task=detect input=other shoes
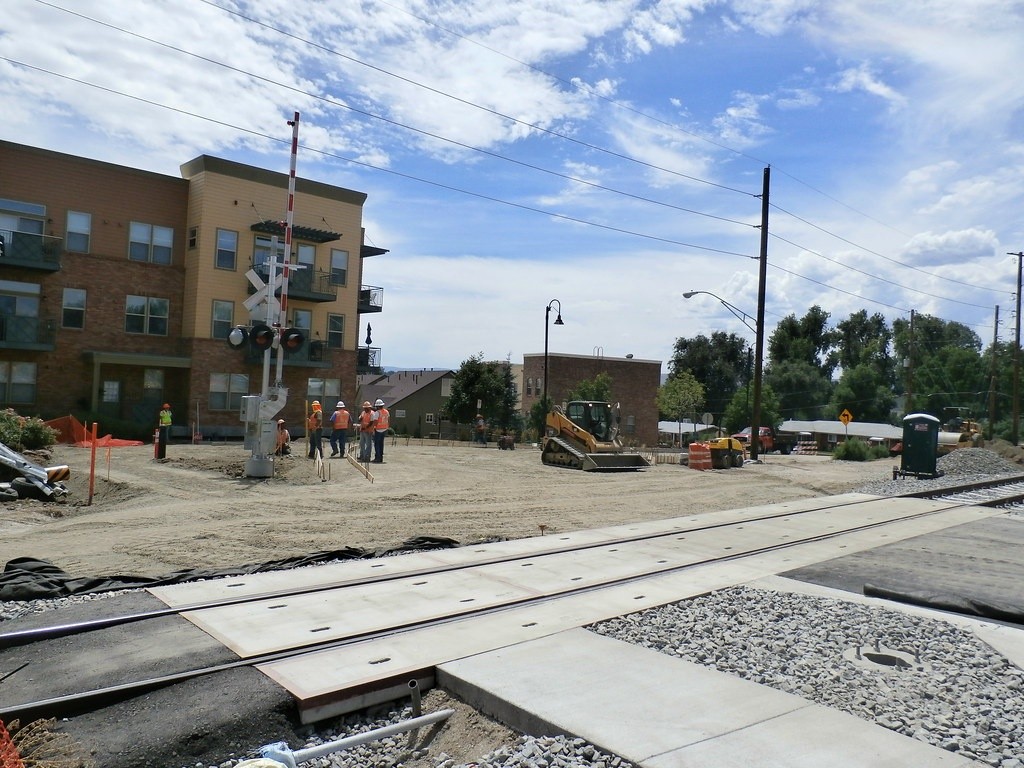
[331,448,338,456]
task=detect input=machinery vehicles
[936,417,985,457]
[540,399,653,472]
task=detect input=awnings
[251,220,342,243]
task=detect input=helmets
[163,403,170,408]
[278,419,285,424]
[335,401,345,407]
[362,401,372,408]
[312,400,321,406]
[374,399,385,406]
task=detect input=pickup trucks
[729,426,797,455]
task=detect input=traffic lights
[250,324,274,351]
[281,327,306,354]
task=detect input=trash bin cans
[892,414,940,480]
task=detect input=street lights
[682,290,765,461]
[541,298,564,437]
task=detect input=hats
[475,414,483,418]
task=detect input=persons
[972,430,981,447]
[474,414,487,444]
[276,419,291,455]
[330,401,353,458]
[308,400,324,459]
[159,404,172,444]
[358,398,391,463]
